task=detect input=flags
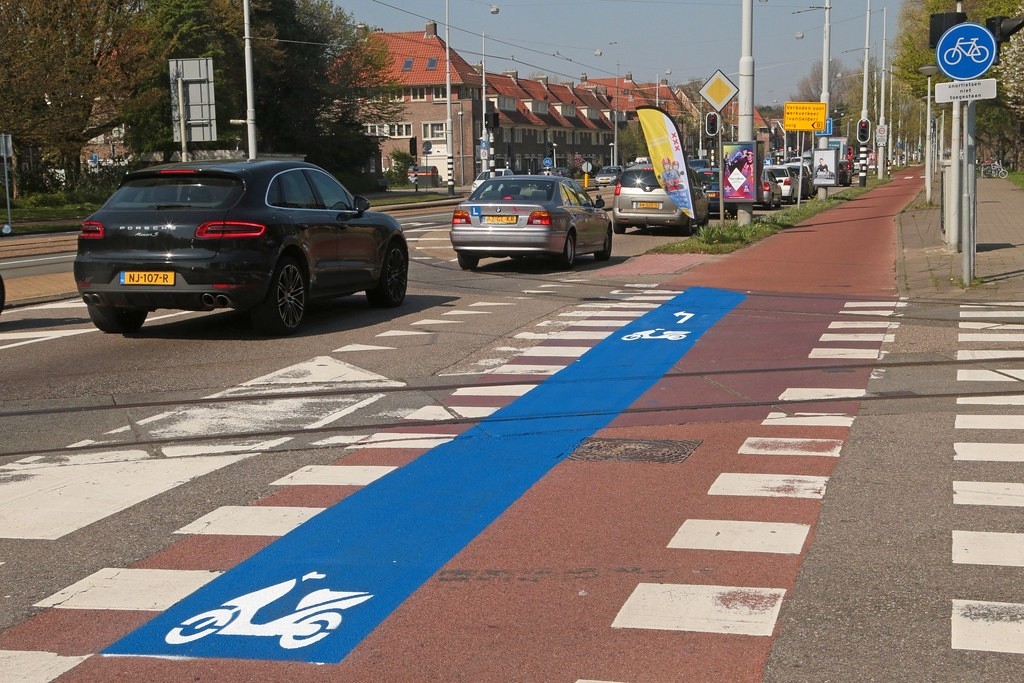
[636,105,695,220]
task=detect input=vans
[634,156,652,165]
[688,159,710,171]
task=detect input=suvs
[73,158,409,336]
[612,164,711,237]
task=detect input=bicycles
[975,156,1010,179]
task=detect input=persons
[817,158,828,172]
[662,157,684,190]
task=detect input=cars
[782,157,818,200]
[594,166,623,188]
[471,168,513,199]
[537,167,575,179]
[839,161,852,187]
[855,157,860,169]
[696,168,737,217]
[763,164,799,205]
[759,168,783,210]
[450,174,613,271]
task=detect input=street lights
[931,102,950,174]
[609,142,614,166]
[918,65,940,205]
[553,143,558,169]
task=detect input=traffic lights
[986,16,1024,42]
[857,118,871,144]
[706,112,719,137]
[847,147,853,158]
